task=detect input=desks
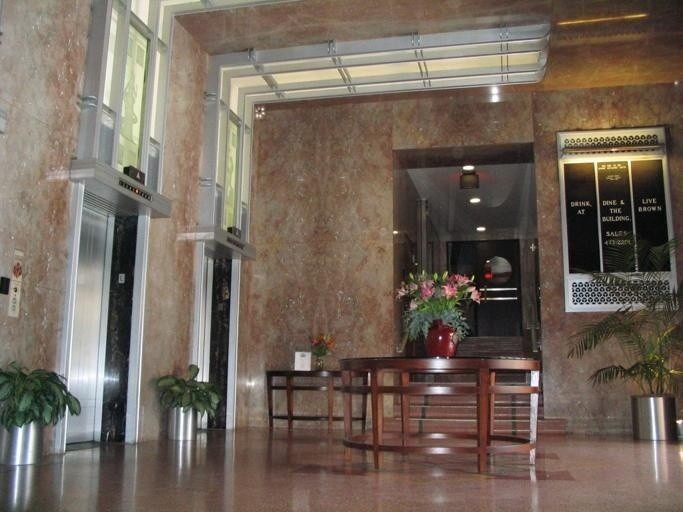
[337,354,547,476]
[261,363,377,435]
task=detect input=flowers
[308,333,335,358]
[394,269,488,345]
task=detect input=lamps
[457,164,482,192]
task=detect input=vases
[313,352,326,372]
[422,317,464,362]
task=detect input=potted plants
[0,360,83,470]
[151,362,222,443]
[560,231,683,444]
